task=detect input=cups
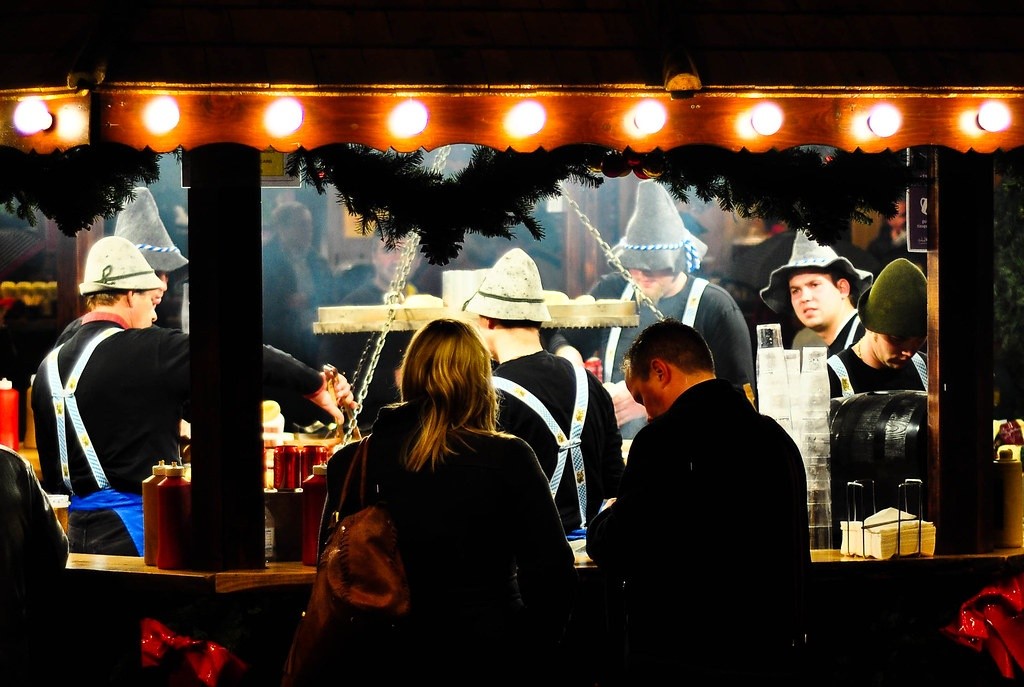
[755,322,832,559]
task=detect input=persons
[585,317,824,687]
[462,247,624,536]
[826,258,927,400]
[0,447,69,687]
[317,320,581,687]
[760,229,873,374]
[548,179,756,439]
[324,230,424,436]
[30,188,359,556]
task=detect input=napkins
[839,506,936,560]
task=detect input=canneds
[273,445,329,493]
[584,357,602,383]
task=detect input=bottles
[142,460,191,570]
[24,374,38,449]
[0,378,19,452]
[993,449,1023,549]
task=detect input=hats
[79,237,168,294]
[857,256,930,334]
[114,187,190,272]
[760,229,873,313]
[462,247,552,324]
[610,179,709,270]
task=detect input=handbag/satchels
[282,505,411,686]
